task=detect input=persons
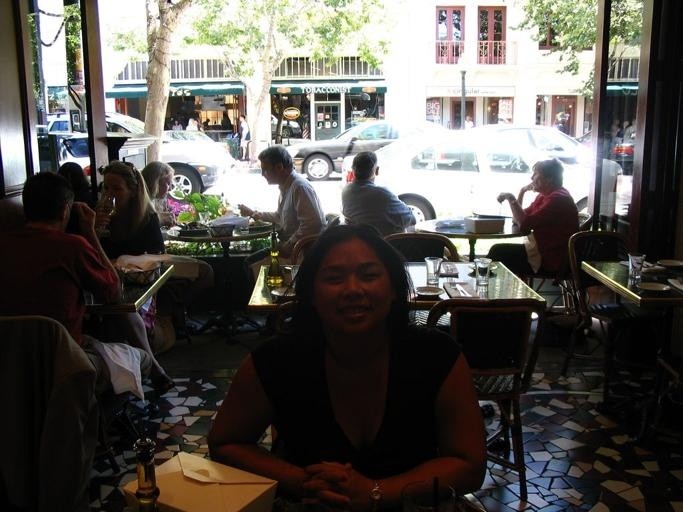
[207,222,488,512]
[341,151,416,239]
[172,120,183,130]
[464,115,474,128]
[237,147,327,314]
[610,118,635,146]
[238,115,250,161]
[0,158,178,428]
[556,119,568,134]
[483,158,579,280]
[185,118,198,131]
[203,119,210,127]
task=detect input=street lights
[458,53,469,129]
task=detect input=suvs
[270,113,302,140]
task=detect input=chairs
[1,211,648,345]
[557,230,668,419]
[427,297,550,502]
[1,314,142,476]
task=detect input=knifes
[455,283,472,297]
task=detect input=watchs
[368,480,383,512]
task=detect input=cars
[47,113,229,200]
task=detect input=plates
[179,230,208,236]
[635,260,683,293]
[468,264,498,271]
[271,288,295,297]
[414,287,444,297]
[249,224,272,231]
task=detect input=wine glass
[95,193,115,239]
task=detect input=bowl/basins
[211,224,235,238]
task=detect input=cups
[424,256,443,284]
[627,253,647,278]
[627,278,642,294]
[198,212,210,226]
[474,258,492,286]
[240,219,249,235]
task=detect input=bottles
[133,438,161,511]
[265,227,284,288]
